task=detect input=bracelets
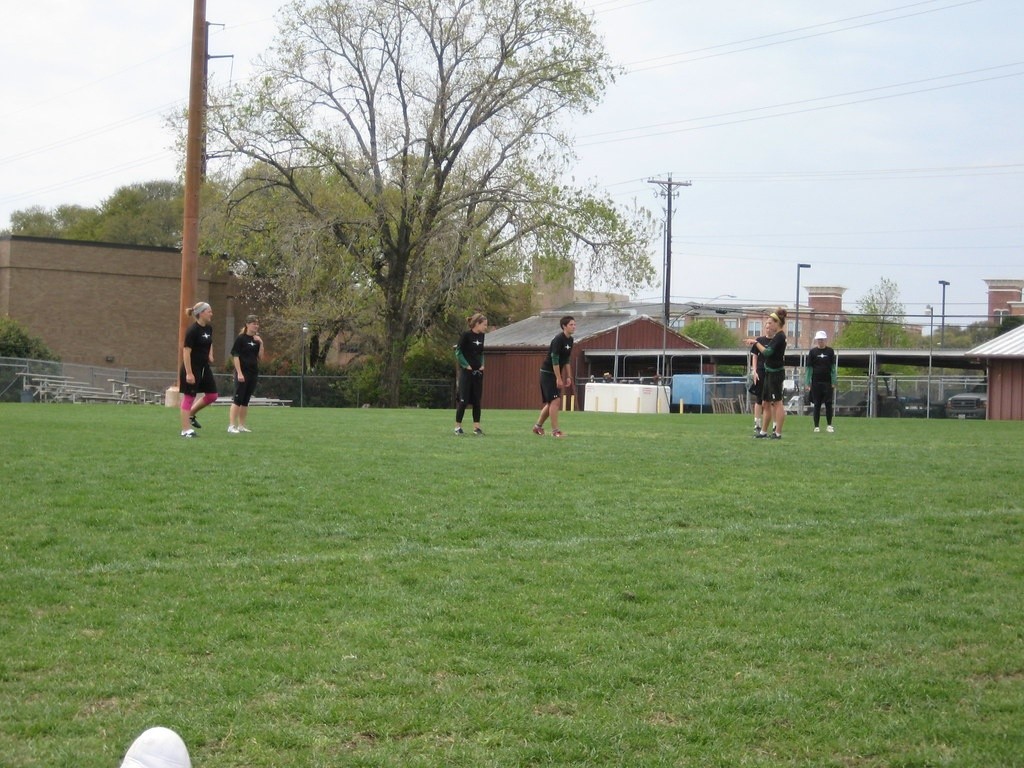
[754,341,758,345]
[567,376,573,378]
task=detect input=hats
[246,315,258,323]
[815,331,827,339]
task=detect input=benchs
[209,399,294,408]
[15,372,165,406]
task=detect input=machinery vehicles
[854,371,933,419]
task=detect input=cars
[944,382,986,420]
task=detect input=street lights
[795,263,812,396]
[938,281,950,400]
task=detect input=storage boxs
[583,383,670,414]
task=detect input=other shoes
[827,426,834,432]
[533,426,544,435]
[455,428,464,435]
[228,425,239,432]
[762,433,781,440]
[814,428,820,432]
[550,431,561,437]
[189,415,201,428]
[753,433,767,439]
[182,429,196,435]
[238,425,251,432]
[473,428,485,435]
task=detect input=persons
[179,302,218,438]
[454,313,488,436]
[120,727,192,768]
[742,307,787,440]
[533,316,575,437]
[227,315,264,434]
[806,331,837,433]
